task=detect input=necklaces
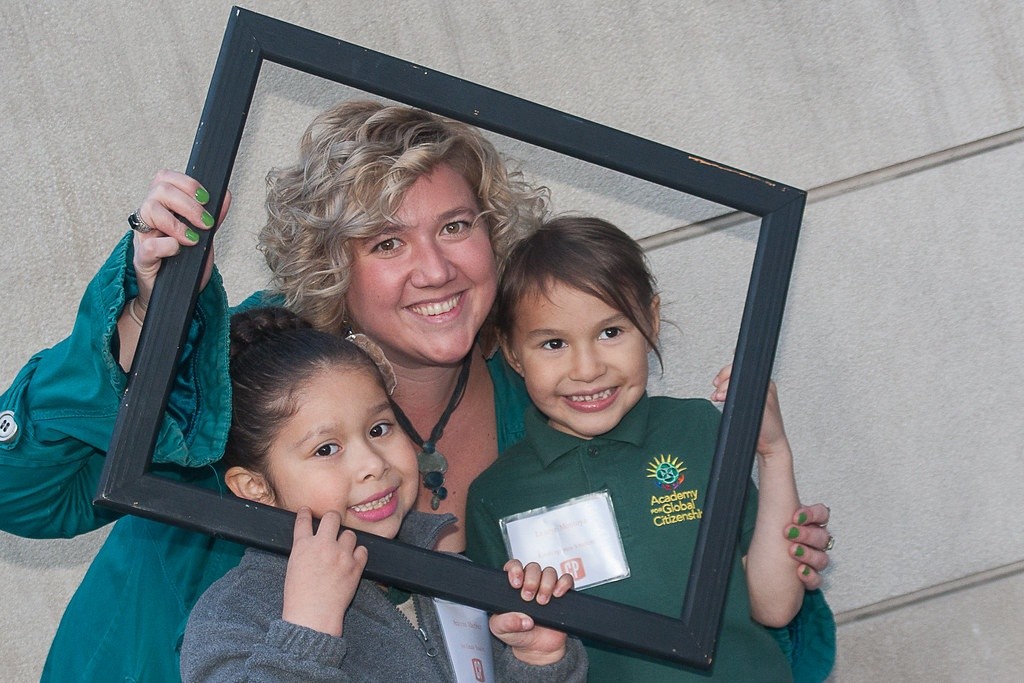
[385,350,472,513]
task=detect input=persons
[1,97,839,683]
[178,305,594,683]
[461,218,811,683]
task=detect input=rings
[821,536,834,552]
[815,506,830,527]
[128,208,156,234]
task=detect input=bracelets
[129,298,146,327]
